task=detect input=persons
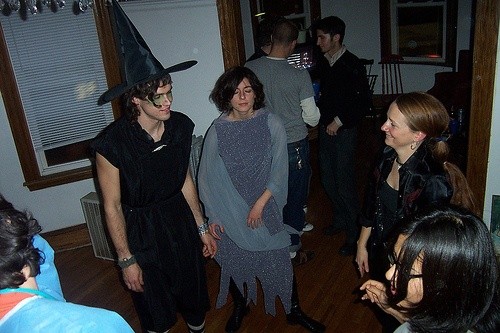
[360,211,500,333]
[199,66,326,333]
[0,193,135,333]
[315,15,373,256]
[92,0,217,333]
[354,92,480,304]
[246,20,321,267]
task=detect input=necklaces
[150,122,161,141]
[396,157,404,166]
[229,111,254,122]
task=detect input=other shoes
[292,250,313,267]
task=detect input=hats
[97,0,197,106]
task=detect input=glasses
[388,249,423,281]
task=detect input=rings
[252,220,254,223]
[358,266,363,270]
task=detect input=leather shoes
[324,223,344,233]
[337,234,359,255]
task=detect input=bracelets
[198,223,209,237]
[118,256,136,269]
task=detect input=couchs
[427,50,471,104]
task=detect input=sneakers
[302,221,313,231]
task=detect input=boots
[286,270,326,333]
[225,277,250,333]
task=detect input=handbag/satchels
[367,229,387,279]
